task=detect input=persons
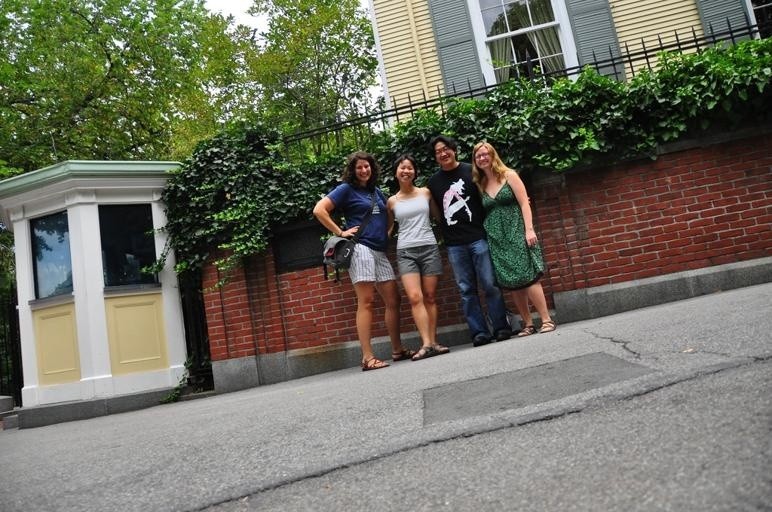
[383,155,450,361]
[424,136,531,346]
[312,151,417,370]
[469,141,556,336]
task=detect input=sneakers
[495,329,511,341]
[471,333,492,347]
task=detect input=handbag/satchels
[323,236,353,269]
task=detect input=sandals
[362,356,390,370]
[518,326,535,336]
[539,321,556,332]
[392,343,449,361]
